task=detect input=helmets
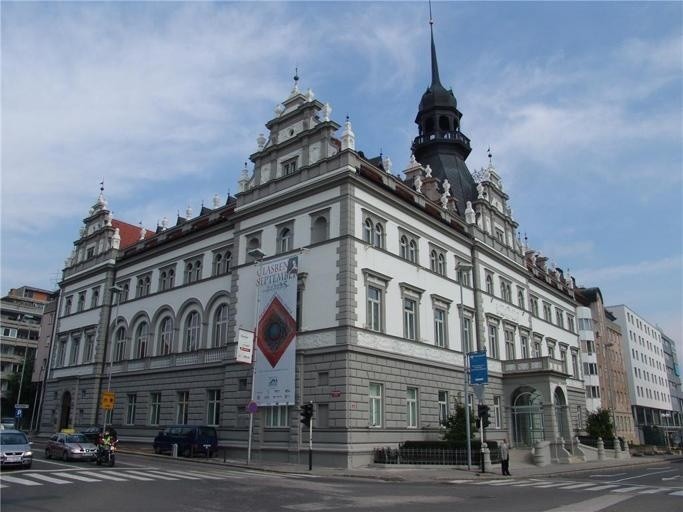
[104,431,109,436]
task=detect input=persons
[500,439,512,476]
[98,431,116,458]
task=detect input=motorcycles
[95,440,118,466]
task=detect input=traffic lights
[301,403,314,426]
[484,406,492,426]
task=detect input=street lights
[604,342,617,437]
[17,314,34,404]
[455,260,473,471]
[248,249,267,461]
[102,285,123,433]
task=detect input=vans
[153,424,218,459]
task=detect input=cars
[0,430,34,468]
[0,417,16,430]
[45,432,99,461]
[73,422,119,445]
